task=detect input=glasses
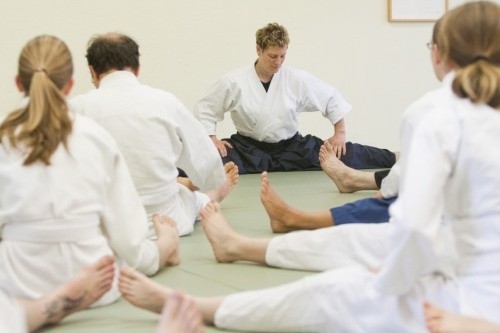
[426,40,433,49]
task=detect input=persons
[177,22,400,177]
[69,31,239,241]
[118,0,499,333]
[423,300,499,333]
[198,14,451,273]
[153,289,205,332]
[0,34,180,308]
[260,157,400,234]
[0,254,114,333]
[317,138,391,194]
